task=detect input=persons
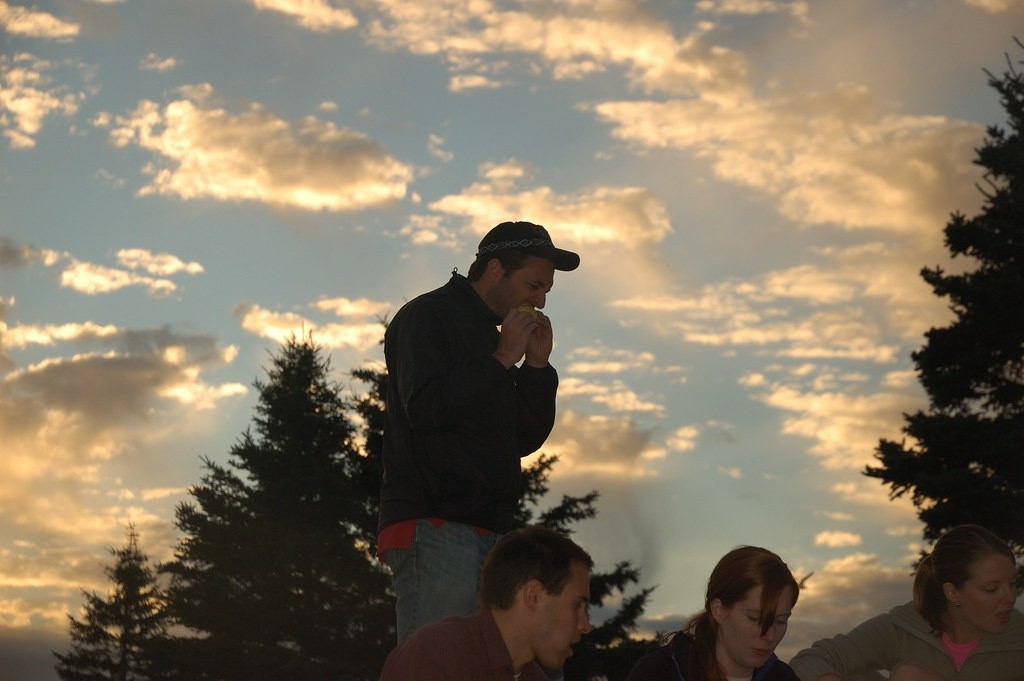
[791,523,1024,681]
[627,546,802,681]
[379,526,597,681]
[376,220,579,681]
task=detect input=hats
[477,221,580,271]
